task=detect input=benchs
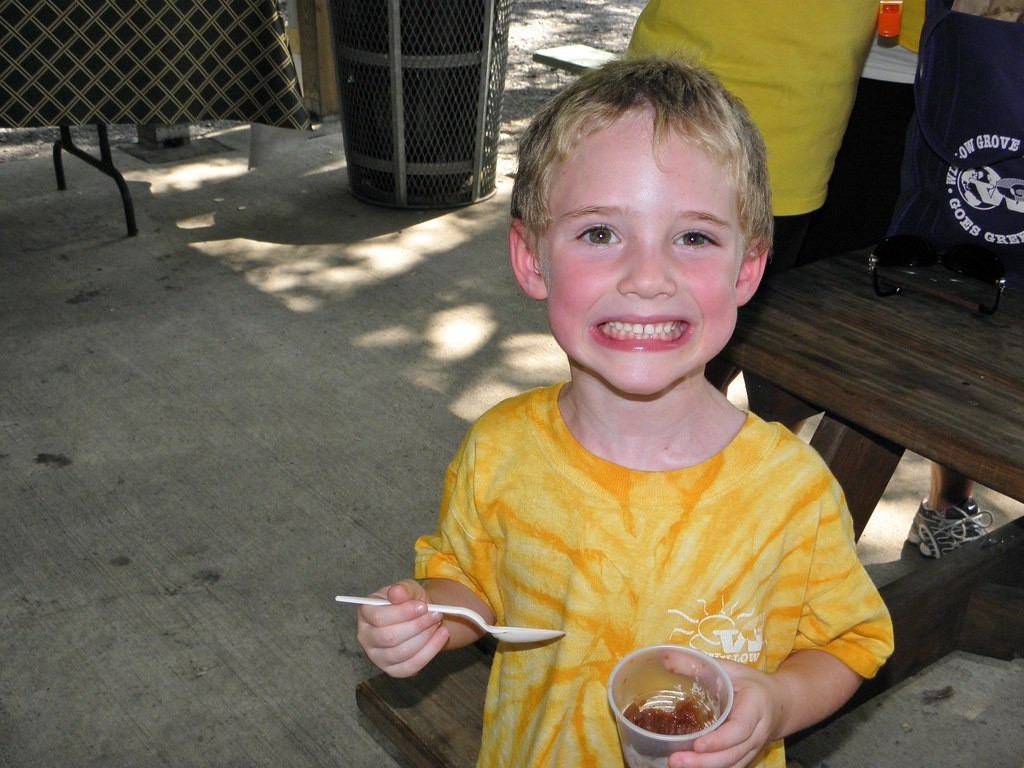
[531,44,619,73]
[356,632,500,768]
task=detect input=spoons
[335,595,566,643]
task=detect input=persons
[628,0,1024,558]
[356,58,894,768]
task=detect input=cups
[608,644,734,768]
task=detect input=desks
[0,0,314,238]
[704,241,1024,747]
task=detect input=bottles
[878,0,903,48]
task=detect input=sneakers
[907,496,993,561]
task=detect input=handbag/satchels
[887,11,1024,279]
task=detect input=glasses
[868,232,1007,316]
